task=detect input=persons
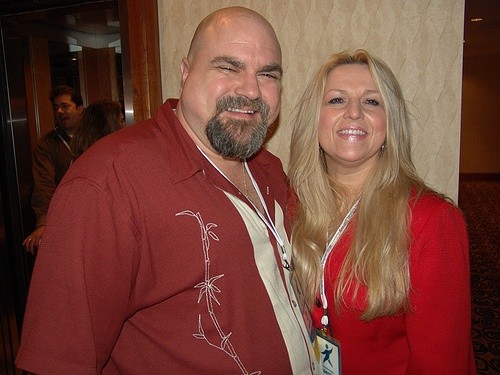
[22,83,127,257]
[14,6,320,375]
[287,49,476,375]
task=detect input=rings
[29,237,31,242]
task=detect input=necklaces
[241,159,250,199]
